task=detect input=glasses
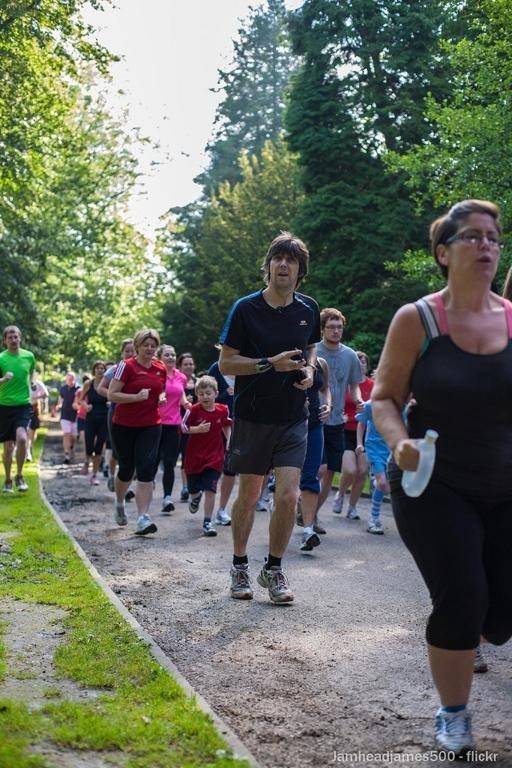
[321,324,346,332]
[439,232,506,252]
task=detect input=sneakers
[0,449,389,537]
[471,645,493,675]
[427,704,479,762]
[254,554,296,605]
[300,524,323,551]
[228,560,256,602]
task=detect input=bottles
[399,429,439,498]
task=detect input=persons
[370,199,512,758]
